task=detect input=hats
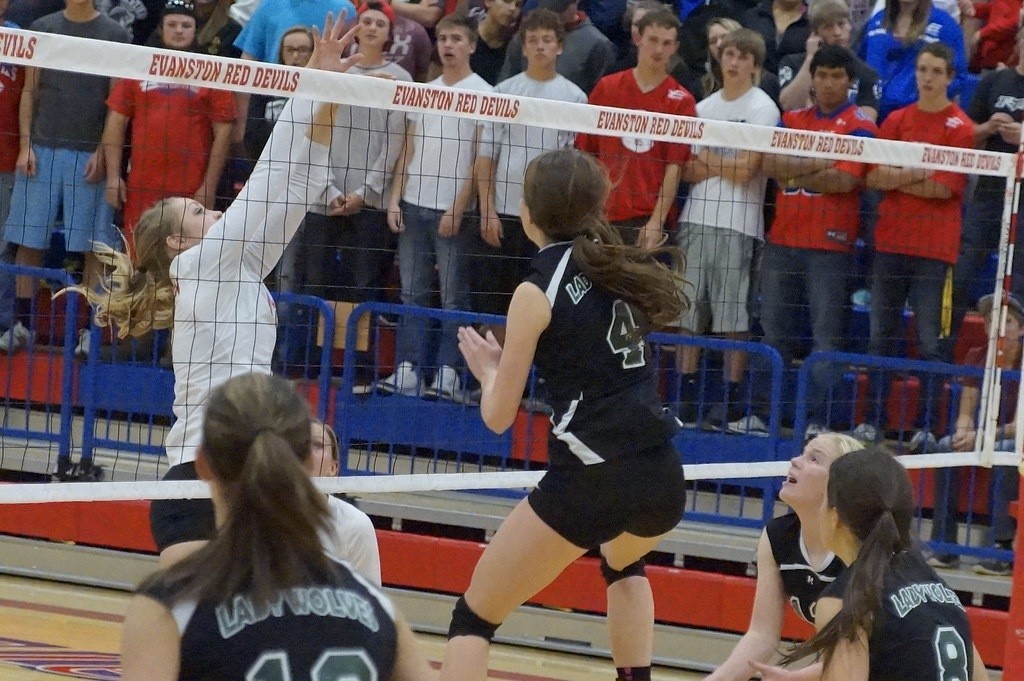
[161,0,199,20]
[979,290,1023,317]
[357,0,396,23]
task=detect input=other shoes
[351,372,375,393]
[727,414,771,438]
[101,334,153,361]
[425,365,459,401]
[804,424,829,441]
[160,344,173,367]
[377,360,425,398]
[665,402,700,429]
[909,429,937,453]
[702,403,729,432]
[852,422,886,447]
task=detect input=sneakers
[0,320,37,355]
[75,329,91,357]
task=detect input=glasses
[283,45,311,57]
[528,35,555,45]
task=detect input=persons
[305,417,381,592]
[52,7,366,568]
[119,371,435,681]
[923,293,1024,577]
[702,432,866,681]
[745,450,994,681]
[0,1,1023,451]
[437,148,692,681]
